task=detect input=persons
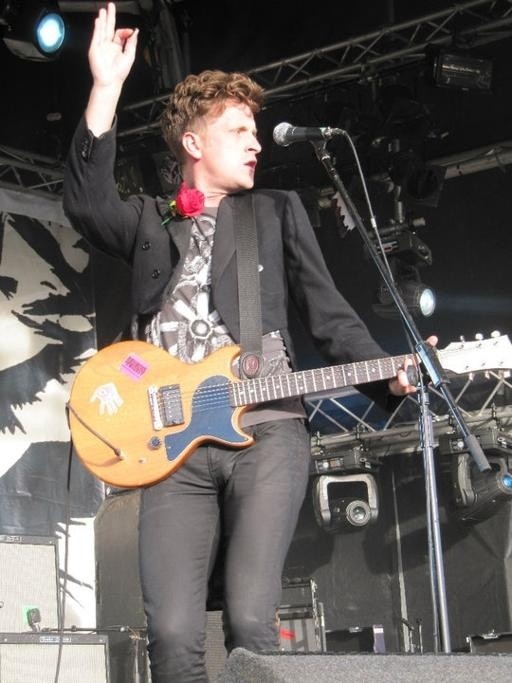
[62,2,438,683]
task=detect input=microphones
[272,122,333,148]
[26,606,42,633]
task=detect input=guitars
[66,331,512,490]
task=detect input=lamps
[1,6,67,62]
[311,473,380,534]
[445,452,511,530]
[372,280,438,321]
